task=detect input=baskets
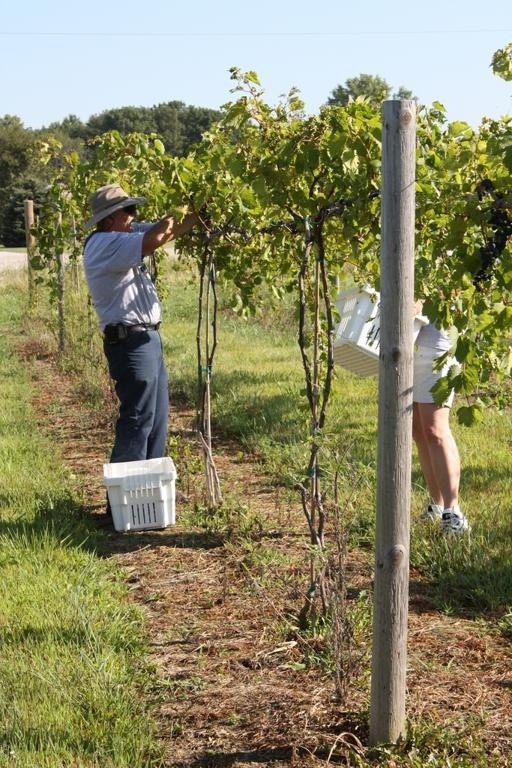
[330,284,430,378]
[102,457,178,534]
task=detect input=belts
[126,322,162,335]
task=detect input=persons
[80,182,202,518]
[414,250,470,534]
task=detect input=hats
[85,183,145,229]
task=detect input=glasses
[121,206,139,215]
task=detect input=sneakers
[421,506,468,538]
